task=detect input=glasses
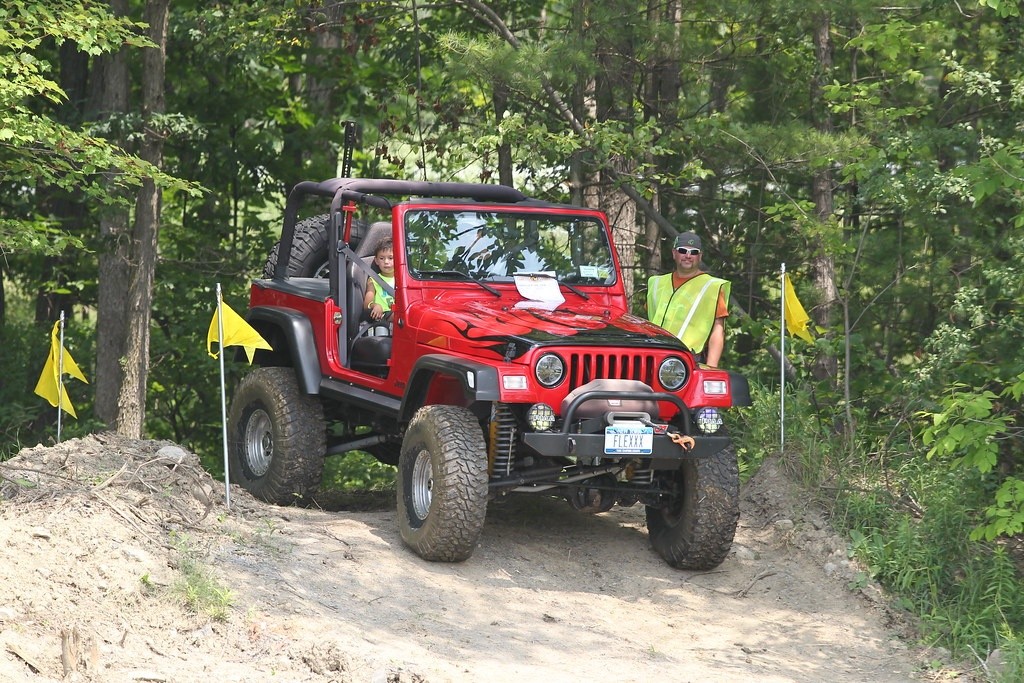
[676,248,700,255]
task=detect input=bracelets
[368,301,374,309]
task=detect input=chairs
[345,222,397,377]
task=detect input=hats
[674,232,701,248]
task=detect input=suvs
[225,178,744,569]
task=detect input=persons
[645,231,732,372]
[363,236,396,329]
[442,212,516,278]
